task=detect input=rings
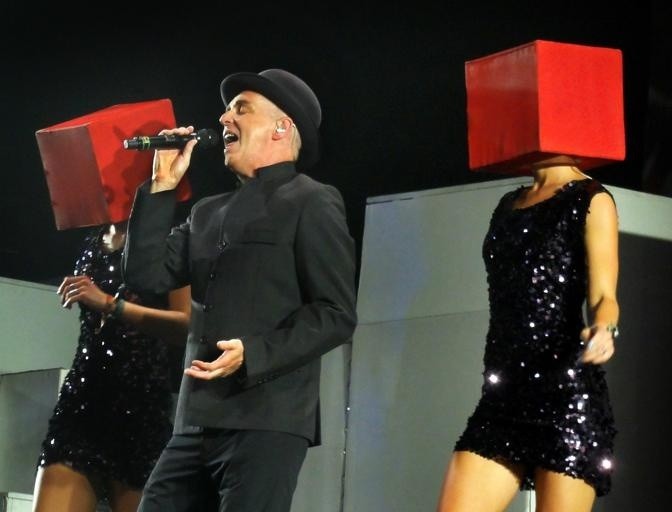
[70,283,76,288]
[69,289,80,295]
[603,351,608,356]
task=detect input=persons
[120,68,359,512]
[30,222,192,512]
[436,154,621,512]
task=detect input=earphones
[276,128,285,133]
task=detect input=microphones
[123,127,220,152]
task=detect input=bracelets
[102,294,113,314]
[606,324,619,340]
[110,298,124,321]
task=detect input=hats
[218,66,325,172]
